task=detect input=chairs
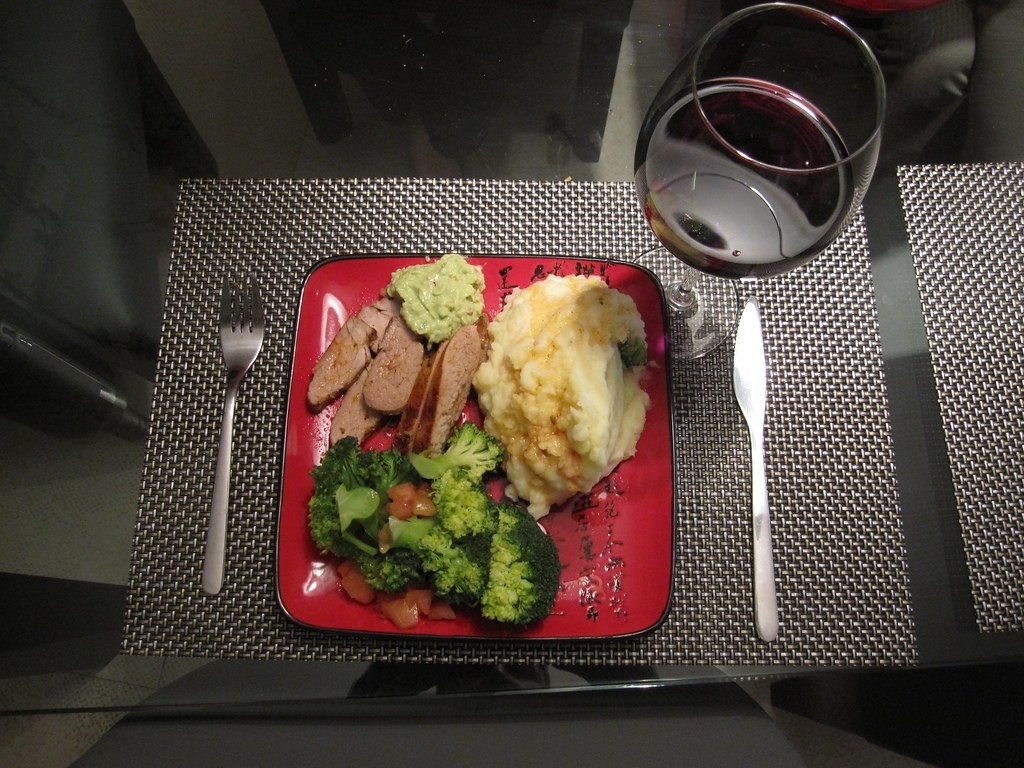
[261,1,633,163]
[0,0,220,438]
[54,655,806,768]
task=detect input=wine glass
[629,3,887,359]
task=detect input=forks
[200,274,265,595]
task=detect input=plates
[273,253,679,639]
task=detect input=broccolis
[308,420,559,630]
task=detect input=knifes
[733,301,781,639]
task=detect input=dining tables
[0,1,1024,716]
